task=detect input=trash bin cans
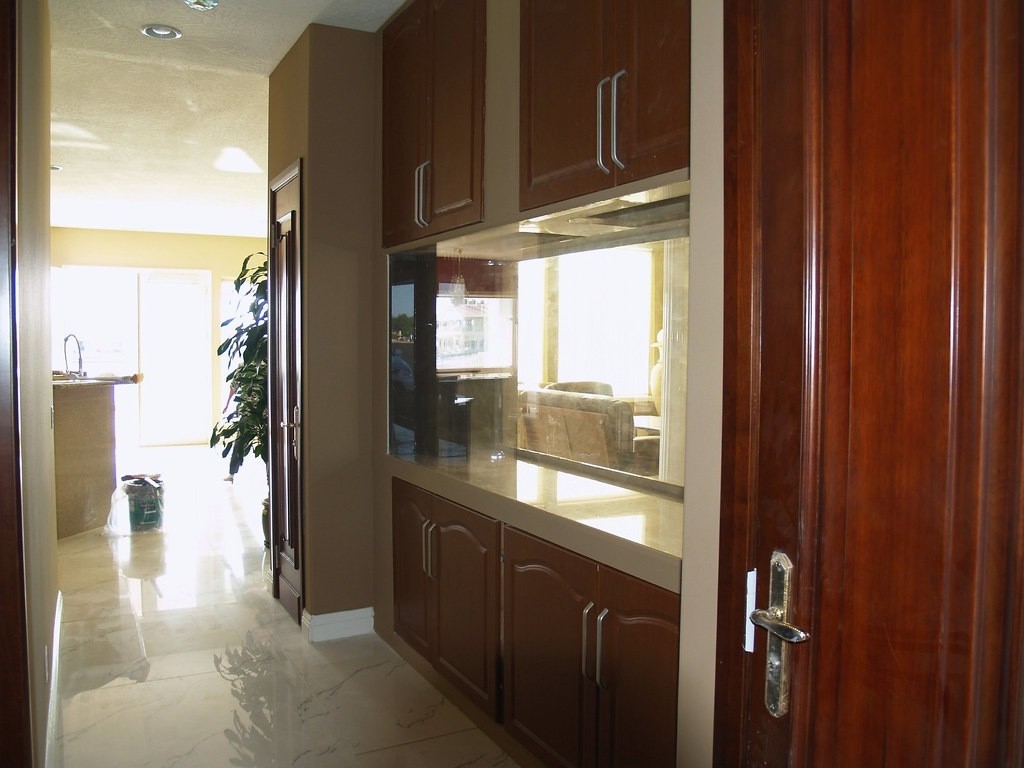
[126,480,164,532]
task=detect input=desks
[633,416,660,432]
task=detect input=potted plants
[211,252,270,549]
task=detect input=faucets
[64,333,82,377]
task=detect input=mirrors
[387,219,690,499]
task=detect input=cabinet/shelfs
[519,0,689,213]
[503,523,680,768]
[393,476,501,725]
[382,0,487,247]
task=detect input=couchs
[538,381,660,477]
